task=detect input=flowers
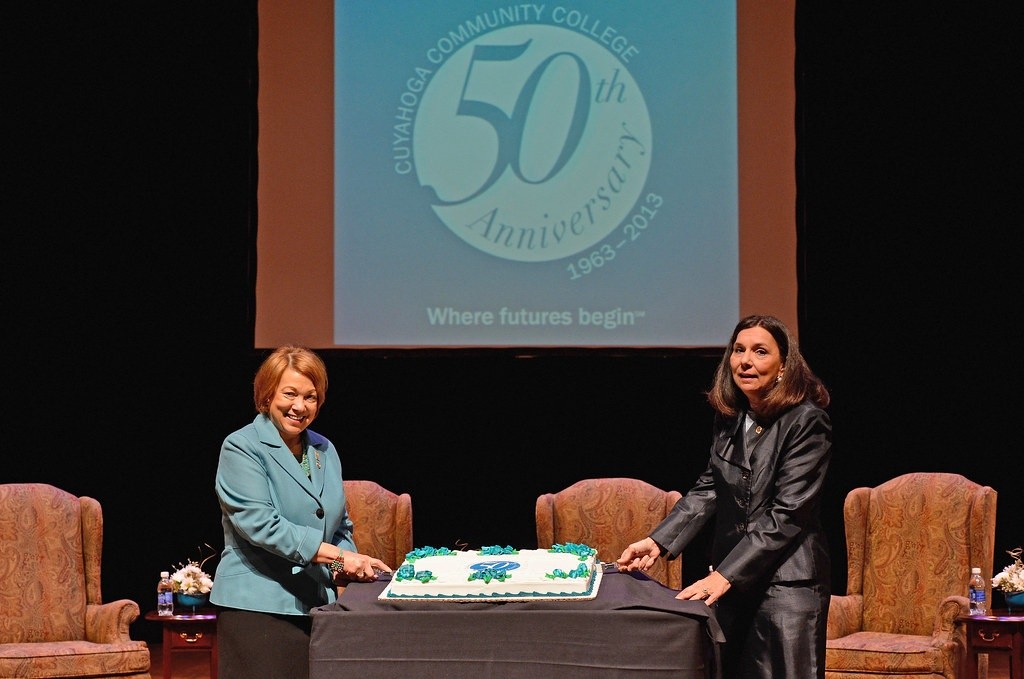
[988,548,1024,593]
[169,555,214,596]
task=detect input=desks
[310,568,725,678]
[959,612,1024,679]
[148,609,223,678]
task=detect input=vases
[176,591,208,614]
[1003,591,1024,615]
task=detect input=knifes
[600,557,634,572]
[371,566,397,577]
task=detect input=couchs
[0,484,153,678]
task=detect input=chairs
[534,476,684,594]
[814,472,995,678]
[334,481,411,594]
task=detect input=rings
[701,588,708,597]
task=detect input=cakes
[387,541,600,597]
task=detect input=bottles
[969,568,986,617]
[158,572,173,617]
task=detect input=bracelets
[331,549,344,573]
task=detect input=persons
[207,345,392,679]
[617,316,834,679]
